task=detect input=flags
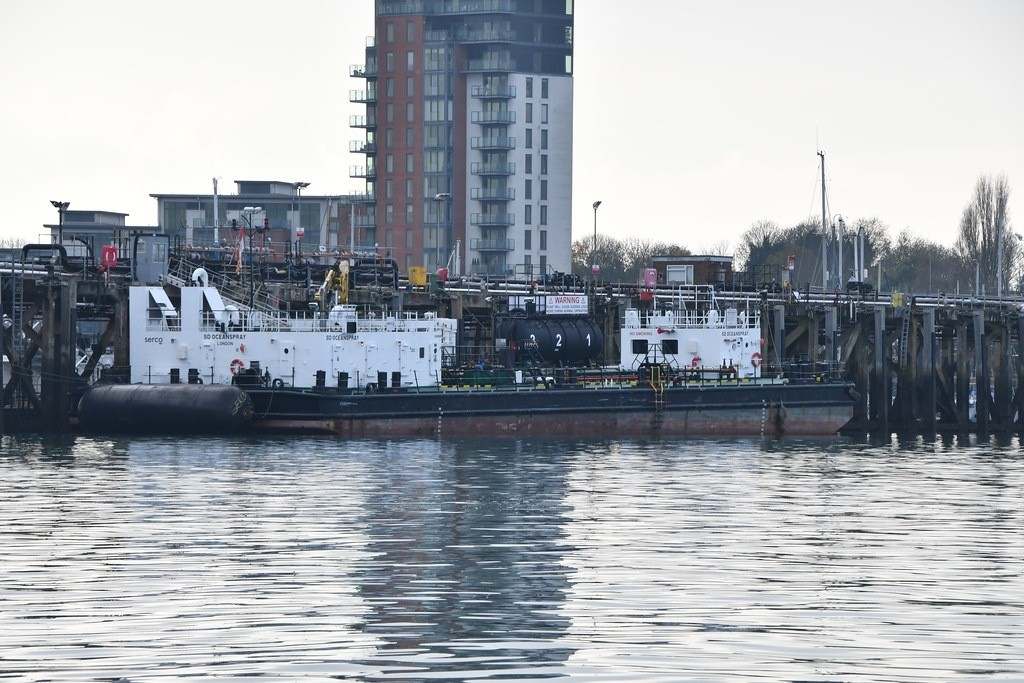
[234,224,244,277]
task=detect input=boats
[76,285,854,436]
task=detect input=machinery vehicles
[308,259,349,310]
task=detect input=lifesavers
[820,373,831,385]
[752,352,762,367]
[272,377,283,389]
[230,359,243,375]
[365,382,377,395]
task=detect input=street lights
[50,200,70,246]
[434,193,450,270]
[593,200,602,265]
[293,182,311,227]
[244,207,262,309]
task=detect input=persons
[771,278,776,293]
[940,291,945,298]
[475,359,485,371]
[834,285,840,292]
